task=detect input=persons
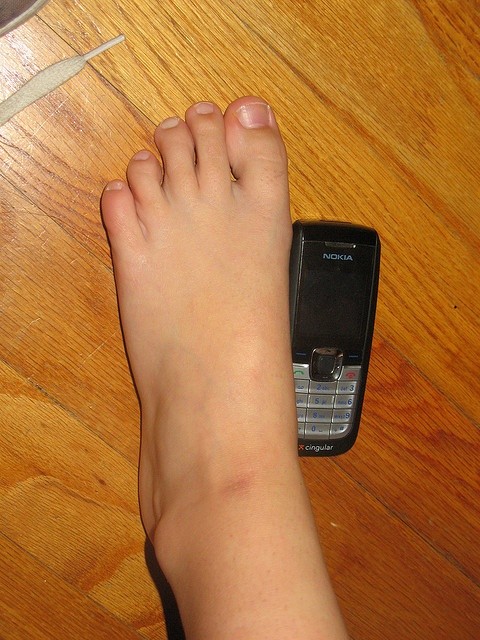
[103,97,349,640]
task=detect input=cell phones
[289,218,381,456]
